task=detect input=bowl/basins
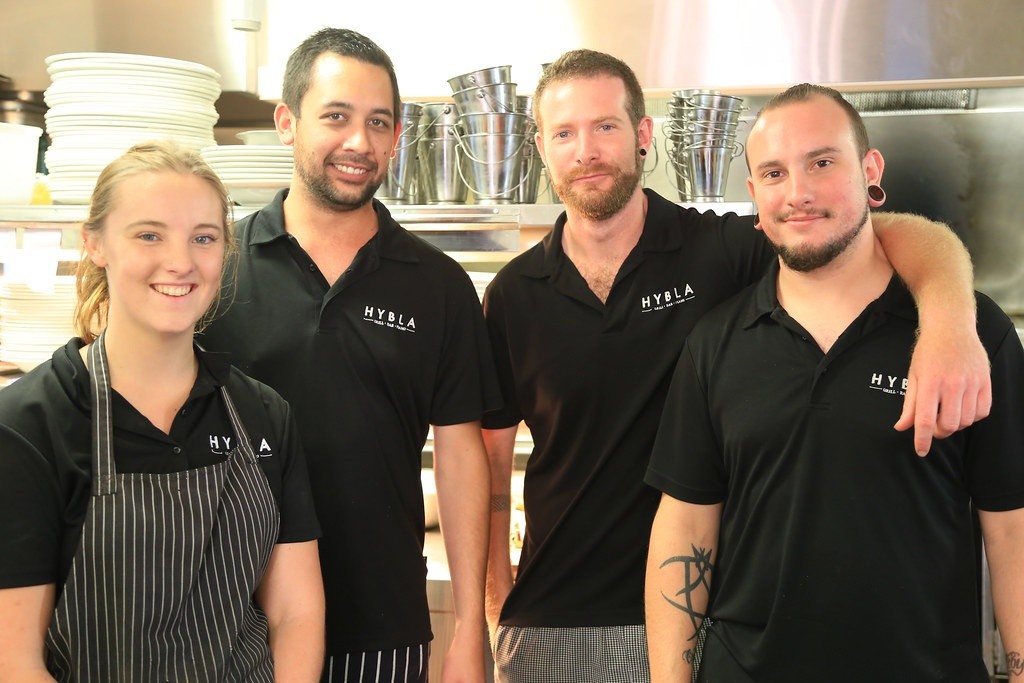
[0,122,43,203]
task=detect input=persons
[0,140,327,683]
[479,48,994,683]
[197,27,493,683]
[643,84,1024,683]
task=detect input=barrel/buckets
[662,87,750,201]
[377,64,563,204]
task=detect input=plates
[0,275,83,364]
[43,52,295,206]
